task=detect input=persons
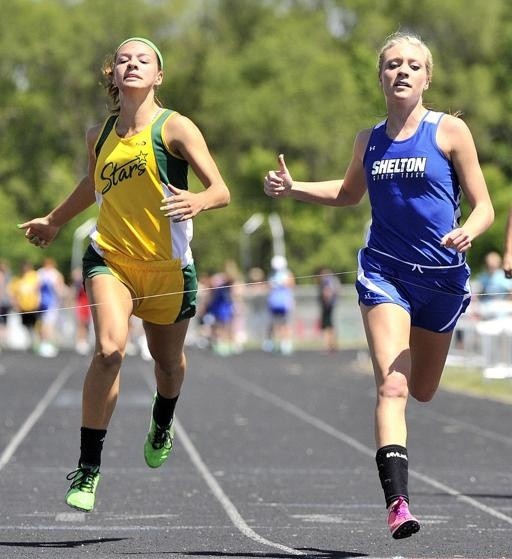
[451,213,511,364]
[184,255,297,356]
[1,256,153,361]
[262,32,496,539]
[312,269,342,358]
[16,36,233,514]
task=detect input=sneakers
[1,330,298,361]
[386,496,420,540]
[64,460,102,514]
[142,390,176,469]
